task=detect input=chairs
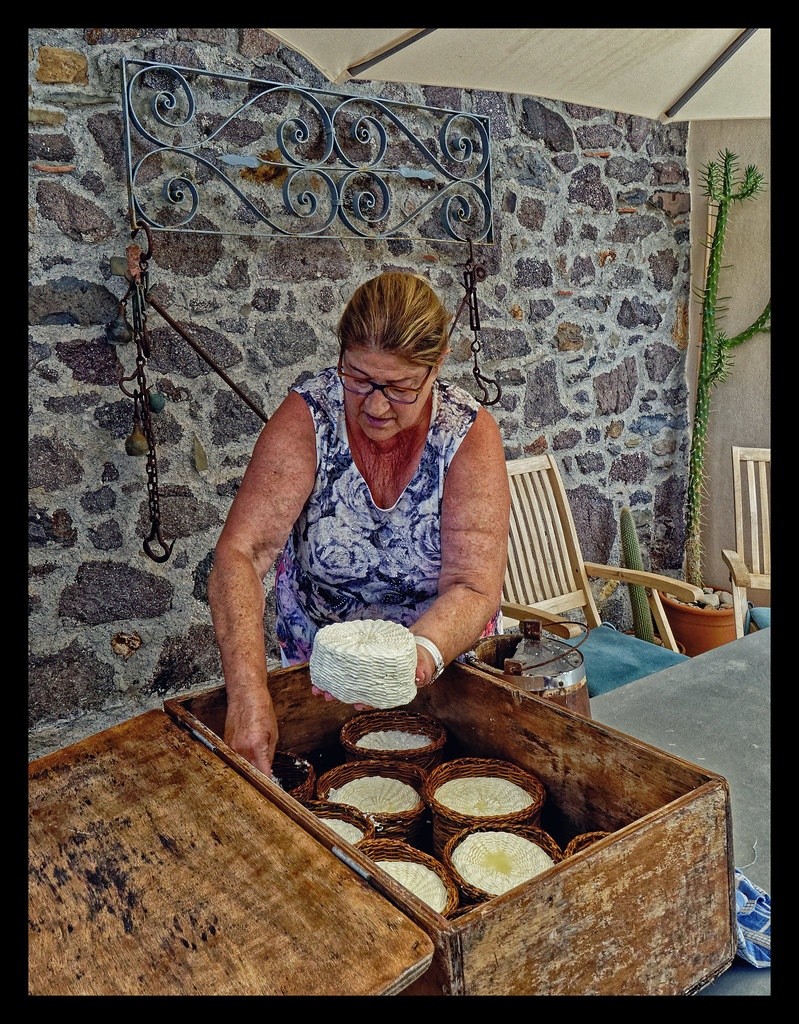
[483,452,700,705]
[718,444,772,639]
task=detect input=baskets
[340,710,446,774]
[351,838,459,918]
[419,758,547,863]
[316,759,430,843]
[297,800,375,845]
[271,749,317,805]
[442,820,565,906]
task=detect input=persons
[206,268,513,781]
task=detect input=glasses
[337,346,433,405]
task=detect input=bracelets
[414,635,445,686]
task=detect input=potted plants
[639,145,772,652]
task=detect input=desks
[586,622,775,994]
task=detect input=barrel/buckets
[464,618,592,718]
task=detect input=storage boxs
[27,636,739,997]
